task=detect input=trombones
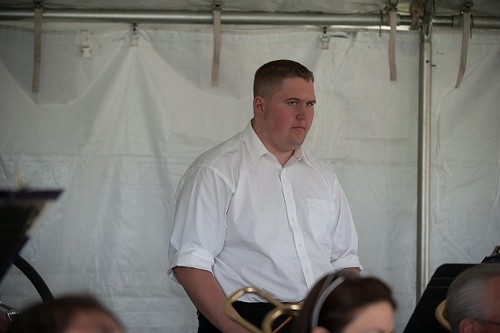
[225,286,304,333]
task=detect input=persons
[1,296,127,333]
[297,272,397,333]
[445,263,500,333]
[166,58,365,333]
